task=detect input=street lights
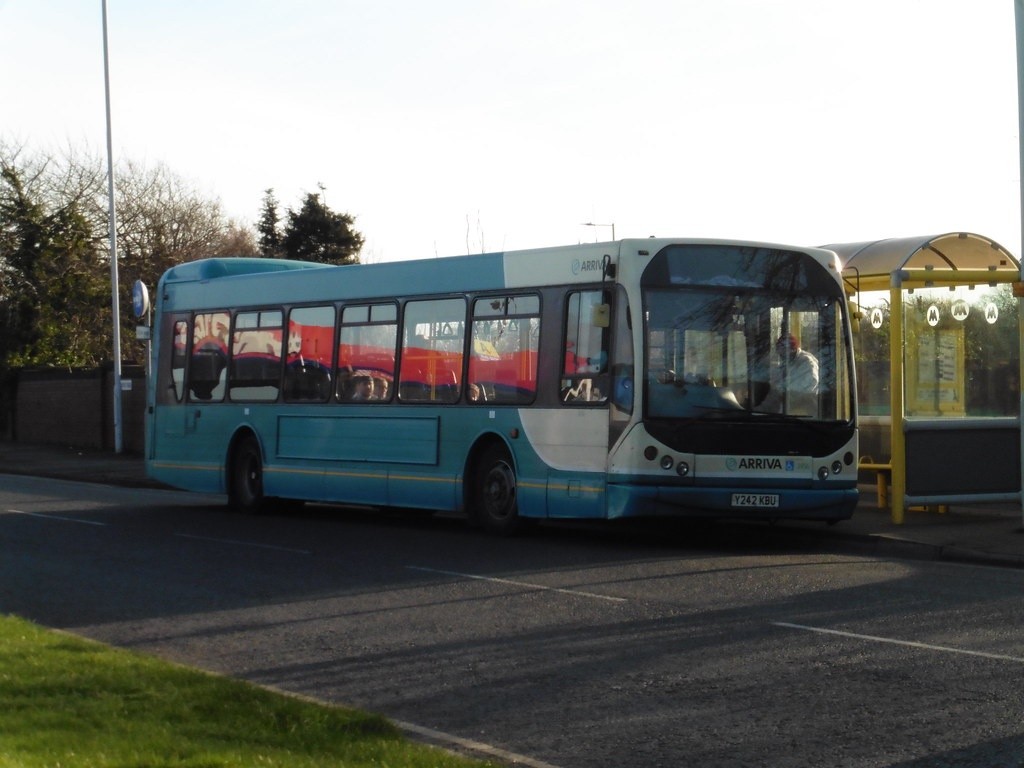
[584,222,615,241]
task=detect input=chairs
[171,347,352,401]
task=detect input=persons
[752,333,819,418]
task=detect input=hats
[775,333,800,350]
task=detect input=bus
[149,242,861,540]
